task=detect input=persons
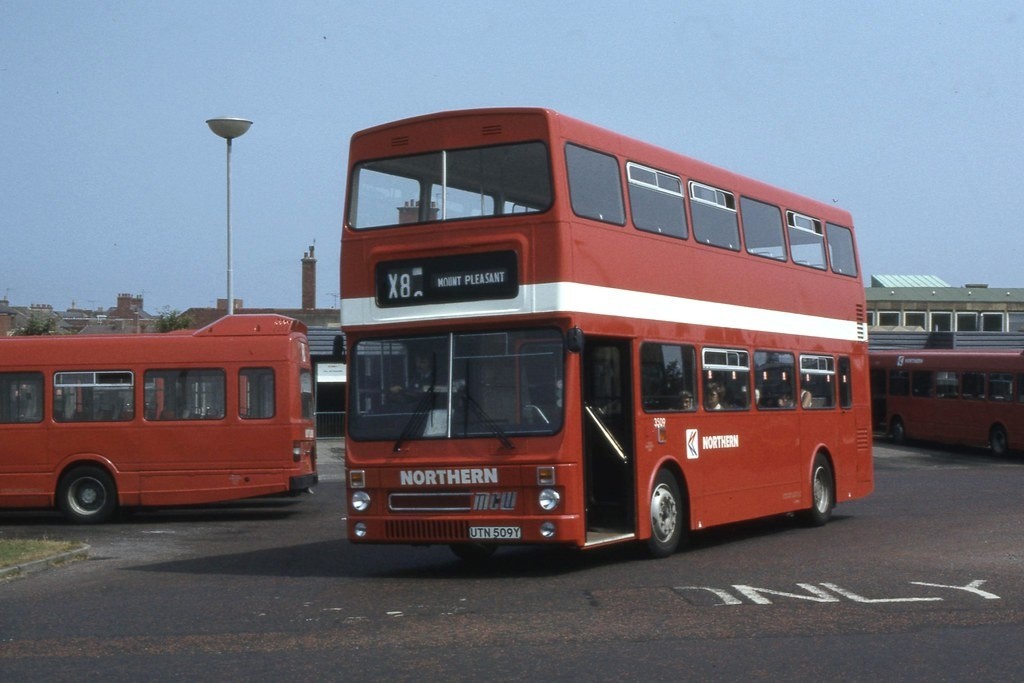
[705,383,726,409]
[676,390,692,410]
[776,375,810,408]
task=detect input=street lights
[205,118,254,315]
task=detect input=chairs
[53,400,259,421]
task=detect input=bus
[0,314,319,526]
[332,107,877,558]
[868,349,1024,459]
